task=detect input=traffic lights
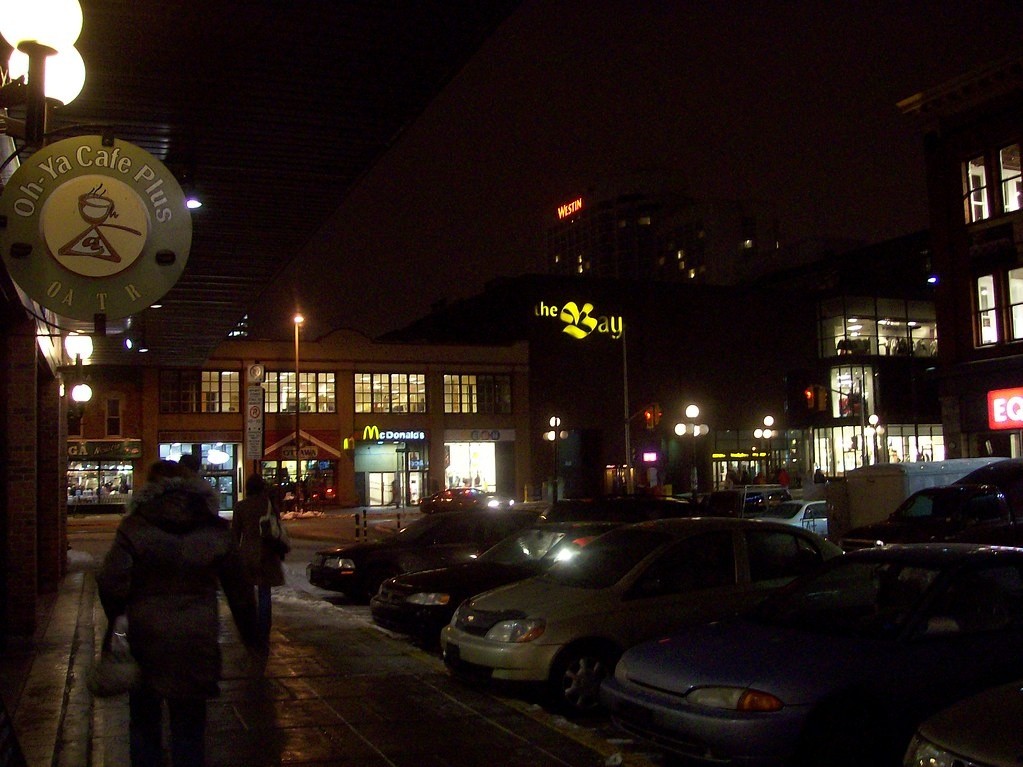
[647,406,653,429]
[806,388,815,409]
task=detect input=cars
[533,459,1023,554]
[370,519,628,632]
[440,515,847,724]
[901,676,1023,767]
[419,489,477,516]
[306,504,539,605]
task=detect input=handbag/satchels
[263,536,288,567]
[85,624,140,698]
[271,566,285,587]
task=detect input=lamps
[124,333,149,354]
[55,329,93,385]
[66,382,92,429]
[0,0,87,149]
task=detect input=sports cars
[597,542,1023,767]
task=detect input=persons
[724,463,767,490]
[97,453,265,767]
[231,472,285,634]
[806,470,814,482]
[885,335,899,356]
[387,477,401,508]
[773,466,789,491]
[814,469,825,484]
[794,471,802,488]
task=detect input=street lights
[864,414,885,464]
[674,404,709,501]
[753,415,779,478]
[293,303,307,479]
[546,416,567,504]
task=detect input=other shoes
[248,669,264,679]
[240,660,256,673]
